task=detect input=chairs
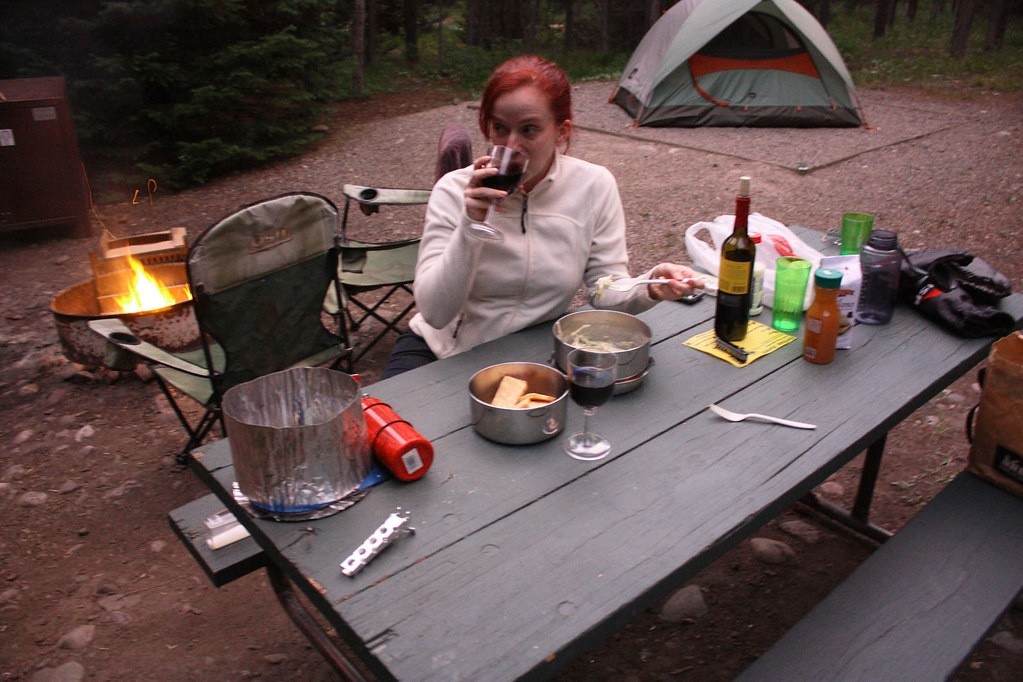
[322,122,478,365]
[88,191,360,465]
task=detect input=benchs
[168,494,270,588]
[728,460,1023,680]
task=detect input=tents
[606,0,880,135]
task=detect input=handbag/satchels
[967,327,1023,502]
[684,211,825,312]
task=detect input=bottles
[714,174,755,341]
[745,232,764,317]
[856,229,904,323]
[348,373,434,478]
[800,269,845,363]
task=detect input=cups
[841,213,874,255]
[772,256,812,331]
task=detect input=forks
[607,275,701,291]
[707,404,817,428]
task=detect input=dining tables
[182,220,1023,682]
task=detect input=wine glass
[561,345,619,460]
[465,144,529,242]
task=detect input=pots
[468,361,567,447]
[547,309,656,392]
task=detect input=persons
[378,52,712,391]
